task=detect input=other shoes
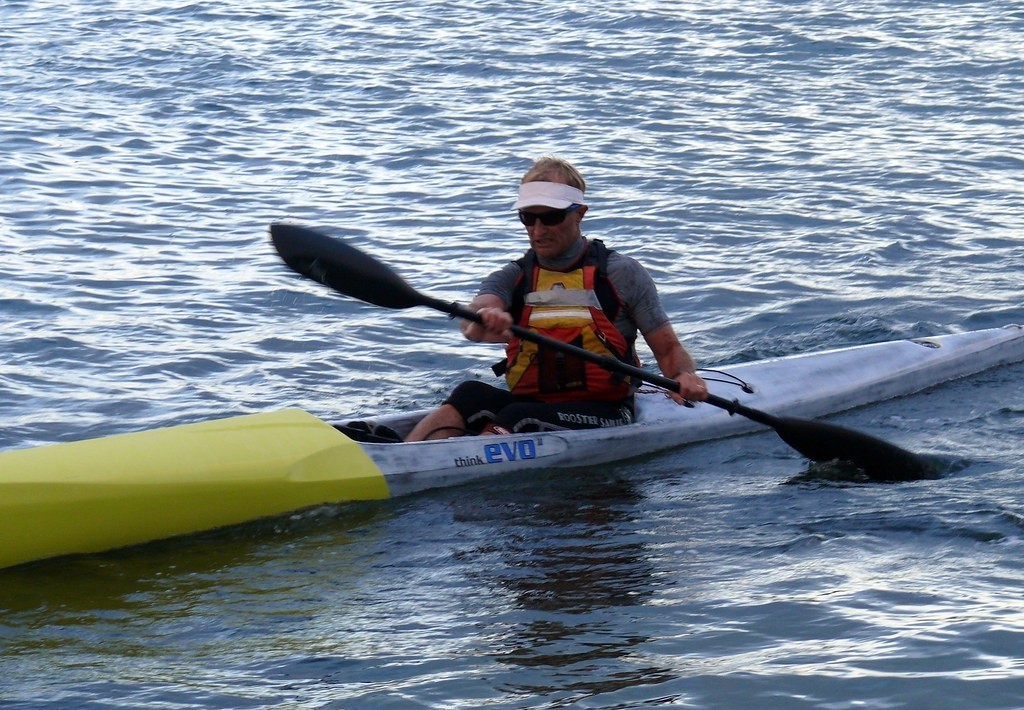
[375,425,403,442]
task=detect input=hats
[511,181,583,209]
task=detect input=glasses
[519,204,582,226]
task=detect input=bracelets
[670,370,695,381]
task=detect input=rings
[697,385,704,388]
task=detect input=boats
[1,319,1024,572]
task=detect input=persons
[348,158,707,442]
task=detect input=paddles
[269,222,943,483]
